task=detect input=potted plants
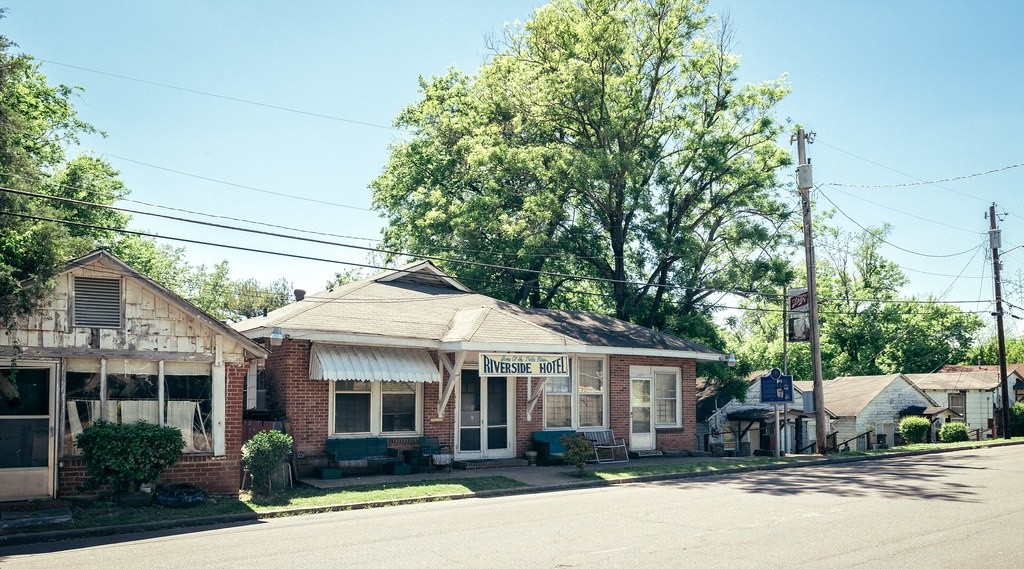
[73,418,187,508]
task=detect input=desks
[402,449,422,474]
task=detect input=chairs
[419,437,452,475]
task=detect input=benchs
[328,438,400,475]
[583,430,629,464]
[531,430,578,466]
